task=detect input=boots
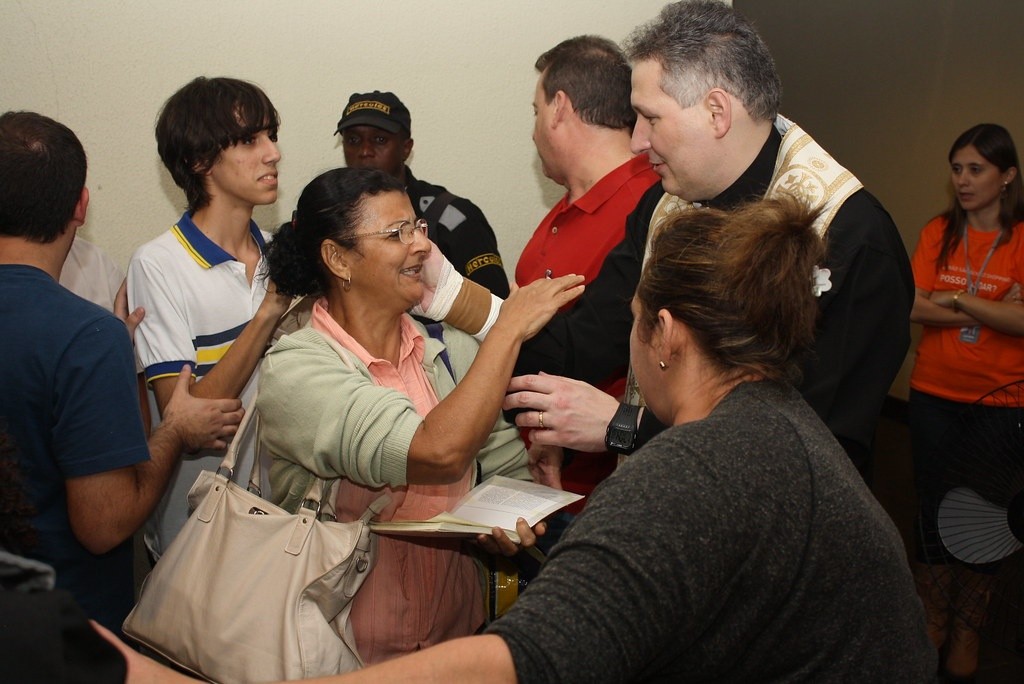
[944,566,1001,676]
[913,562,956,649]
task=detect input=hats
[334,90,410,138]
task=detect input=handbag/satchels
[122,330,392,684]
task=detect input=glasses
[338,219,428,245]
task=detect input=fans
[917,379,1024,662]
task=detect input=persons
[58,236,151,446]
[253,168,565,667]
[280,187,937,684]
[408,0,916,474]
[335,89,513,300]
[515,31,661,599]
[122,75,296,572]
[0,430,127,684]
[910,123,1024,684]
[0,110,246,647]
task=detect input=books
[370,475,586,545]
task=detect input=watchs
[605,401,643,454]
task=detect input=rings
[538,411,545,428]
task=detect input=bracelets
[953,289,967,311]
